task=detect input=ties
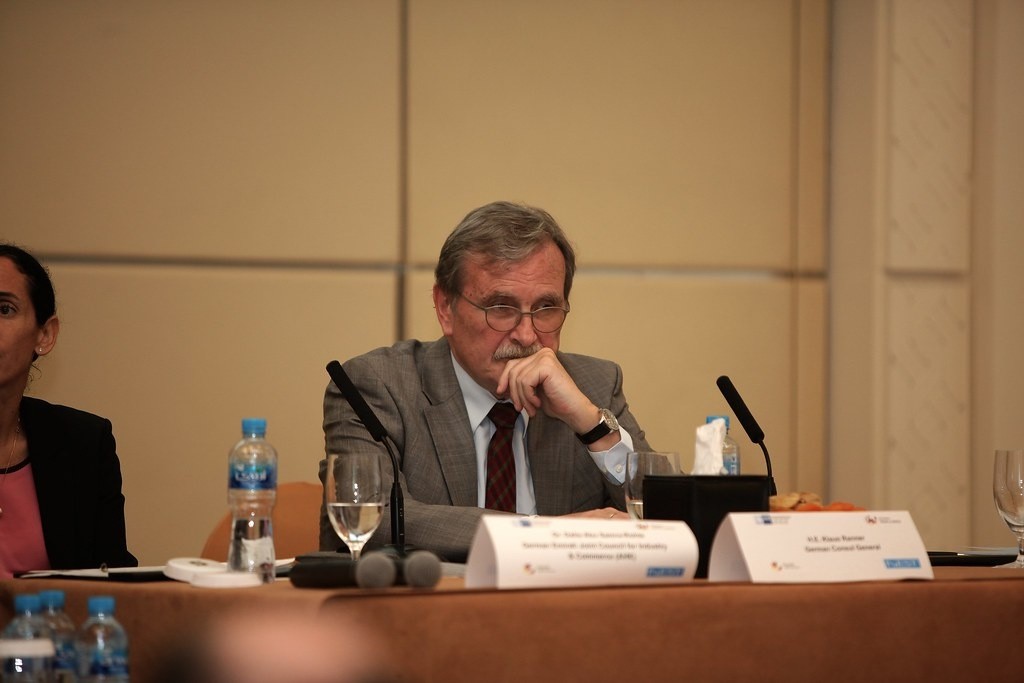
[485,401,521,515]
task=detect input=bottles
[38,589,77,683]
[225,417,278,582]
[705,415,742,476]
[75,595,131,683]
[0,593,59,683]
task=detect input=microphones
[289,359,443,591]
[716,375,773,497]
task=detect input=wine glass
[991,448,1024,569]
[323,450,387,562]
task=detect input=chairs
[199,484,322,562]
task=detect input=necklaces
[0,417,19,514]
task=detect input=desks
[0,562,1024,683]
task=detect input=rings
[609,513,615,518]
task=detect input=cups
[625,450,682,521]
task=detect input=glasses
[454,286,571,333]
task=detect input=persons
[0,244,138,581]
[323,202,675,561]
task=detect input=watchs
[575,407,620,446]
